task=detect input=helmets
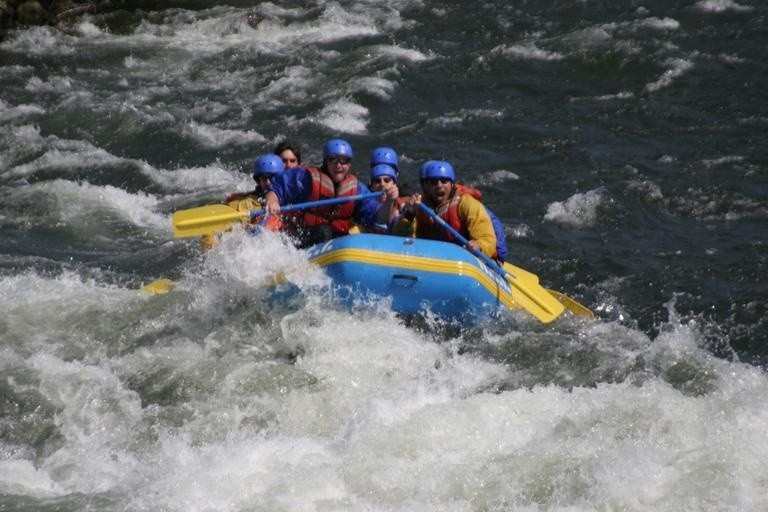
[425,161,455,181]
[322,139,353,160]
[369,164,398,185]
[419,160,437,180]
[370,147,399,168]
[253,154,285,178]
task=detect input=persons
[265,139,399,248]
[363,145,436,239]
[224,152,285,236]
[404,160,508,268]
[275,138,301,170]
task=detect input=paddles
[413,196,564,323]
[478,256,594,319]
[172,185,393,239]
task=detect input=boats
[133,191,597,327]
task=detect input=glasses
[374,177,392,184]
[425,178,450,186]
[327,157,350,164]
[257,175,277,185]
